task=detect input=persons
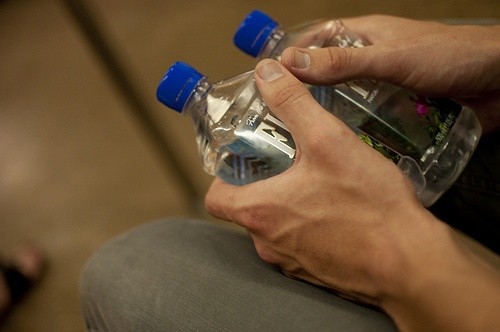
[80,13,500,332]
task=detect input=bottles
[233,10,482,207]
[156,61,425,195]
